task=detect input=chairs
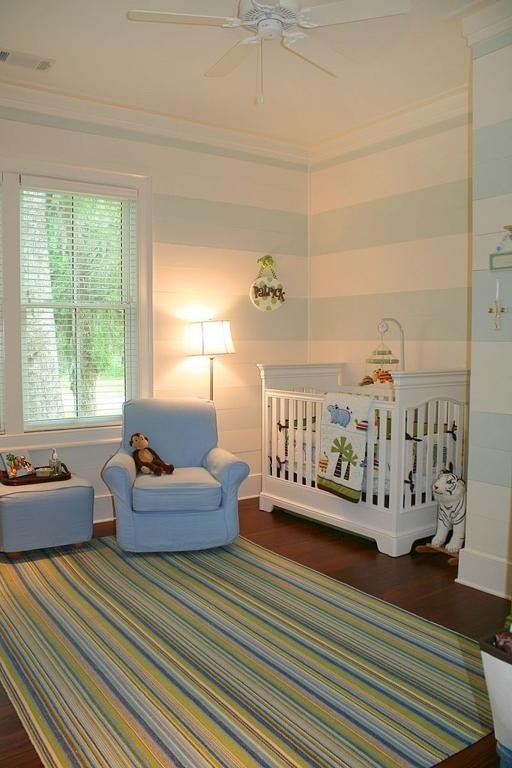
[100,398,251,553]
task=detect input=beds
[256,363,470,558]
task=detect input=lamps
[182,319,236,401]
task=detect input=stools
[0,474,94,560]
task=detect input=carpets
[0,534,495,768]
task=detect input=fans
[126,0,410,105]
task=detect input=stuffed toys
[372,367,393,383]
[129,431,174,475]
[357,375,373,386]
